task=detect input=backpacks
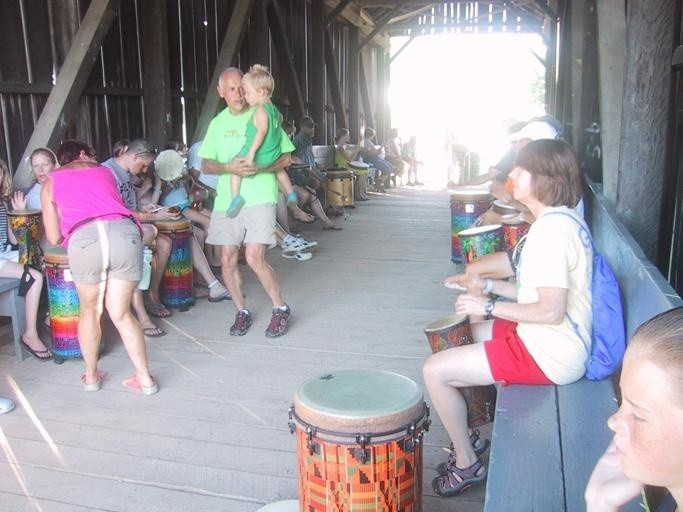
[542,212,625,382]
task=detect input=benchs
[312,143,379,174]
[482,170,683,511]
[0,269,42,363]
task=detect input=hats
[299,116,318,127]
[509,120,558,142]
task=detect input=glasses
[136,147,158,155]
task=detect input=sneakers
[230,309,253,336]
[283,236,318,255]
[357,196,367,201]
[286,191,297,210]
[282,252,313,261]
[226,195,245,218]
[362,194,368,200]
[265,302,293,338]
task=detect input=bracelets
[484,278,493,293]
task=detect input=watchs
[486,300,496,318]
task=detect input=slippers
[322,224,343,231]
[293,214,316,224]
[81,369,105,392]
[121,374,160,396]
[208,288,246,303]
[146,303,172,318]
[21,335,54,361]
[143,326,167,338]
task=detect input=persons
[277,119,345,260]
[226,64,298,219]
[384,128,409,186]
[363,128,393,192]
[196,67,297,337]
[1,138,248,416]
[584,306,682,512]
[423,138,594,499]
[466,121,593,323]
[333,128,371,201]
[446,132,471,187]
[403,136,424,186]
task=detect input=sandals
[436,430,489,476]
[432,455,487,498]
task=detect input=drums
[43,246,105,360]
[289,164,311,186]
[6,208,43,265]
[295,368,422,512]
[349,161,369,176]
[326,170,353,206]
[154,149,187,182]
[423,313,497,428]
[151,217,195,309]
[188,183,205,210]
[450,190,531,266]
